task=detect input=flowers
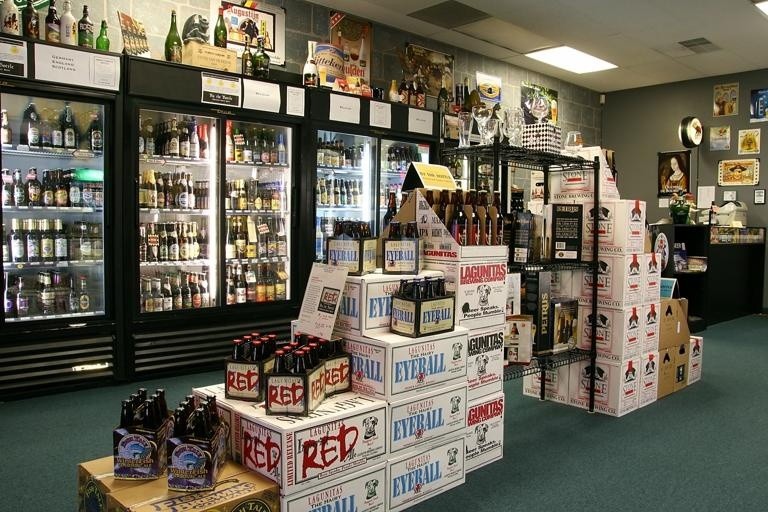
[667,191,694,206]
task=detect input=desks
[651,225,766,330]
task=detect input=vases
[669,205,690,225]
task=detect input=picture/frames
[657,150,691,207]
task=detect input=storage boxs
[340,269,441,334]
[575,307,659,361]
[79,455,169,511]
[676,299,690,345]
[550,146,618,199]
[575,253,661,310]
[522,123,560,154]
[527,170,544,201]
[415,187,508,263]
[422,260,507,326]
[658,299,677,347]
[106,461,280,512]
[468,325,504,403]
[196,383,266,461]
[661,347,674,400]
[239,392,385,495]
[279,461,387,512]
[570,357,660,417]
[467,392,503,475]
[347,327,469,400]
[676,345,689,395]
[691,335,702,387]
[384,382,469,453]
[290,321,345,345]
[383,431,465,512]
[522,357,569,406]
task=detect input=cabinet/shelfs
[442,134,599,417]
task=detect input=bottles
[52,116,62,147]
[147,170,157,208]
[398,79,408,105]
[243,130,253,161]
[173,393,218,438]
[359,36,366,67]
[54,168,67,206]
[187,173,195,208]
[200,218,209,259]
[303,42,318,87]
[246,222,257,258]
[68,167,83,206]
[80,221,91,259]
[408,81,416,105]
[492,191,503,245]
[27,102,39,146]
[276,215,287,256]
[232,332,276,362]
[82,168,93,206]
[388,220,418,240]
[316,177,362,206]
[53,272,65,313]
[278,133,285,163]
[140,265,210,312]
[145,117,155,154]
[41,170,54,206]
[463,77,470,111]
[16,277,29,317]
[267,217,276,257]
[40,273,55,315]
[256,264,266,302]
[160,120,167,155]
[7,218,23,262]
[234,128,243,161]
[388,80,398,102]
[95,182,103,207]
[253,36,270,79]
[317,138,363,168]
[168,117,179,156]
[120,387,168,429]
[0,0,19,35]
[12,168,25,205]
[270,128,278,163]
[214,6,227,48]
[380,183,402,207]
[71,282,71,283]
[568,309,577,338]
[60,1,78,45]
[315,217,328,260]
[456,83,463,105]
[253,127,260,162]
[478,190,492,245]
[68,274,80,311]
[261,127,270,162]
[334,217,342,237]
[147,222,200,260]
[179,125,190,156]
[257,217,267,258]
[344,41,350,65]
[2,224,9,262]
[398,275,446,299]
[450,189,467,246]
[4,274,16,317]
[242,35,253,76]
[37,272,44,309]
[557,311,565,343]
[416,82,425,107]
[138,115,145,153]
[225,127,234,161]
[383,192,396,231]
[40,219,53,261]
[45,0,61,42]
[247,265,255,303]
[70,221,80,259]
[387,146,421,171]
[26,167,41,205]
[469,189,481,246]
[234,216,246,259]
[400,192,408,209]
[78,5,94,48]
[510,323,519,345]
[62,106,75,149]
[165,118,172,155]
[232,216,237,236]
[165,172,173,207]
[200,124,210,158]
[179,172,188,209]
[42,120,51,146]
[437,189,448,224]
[92,224,102,260]
[195,181,209,209]
[54,219,68,261]
[22,0,39,38]
[234,265,247,304]
[273,330,329,375]
[79,275,90,311]
[24,218,40,262]
[225,178,287,211]
[96,20,110,51]
[90,114,103,150]
[174,173,180,205]
[165,10,182,63]
[276,262,286,301]
[138,174,147,208]
[139,223,147,261]
[226,266,235,305]
[709,201,717,224]
[265,264,275,301]
[157,171,165,207]
[1,168,11,205]
[226,215,236,259]
[0,109,12,144]
[189,116,200,158]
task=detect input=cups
[472,106,491,146]
[478,120,498,144]
[458,112,473,148]
